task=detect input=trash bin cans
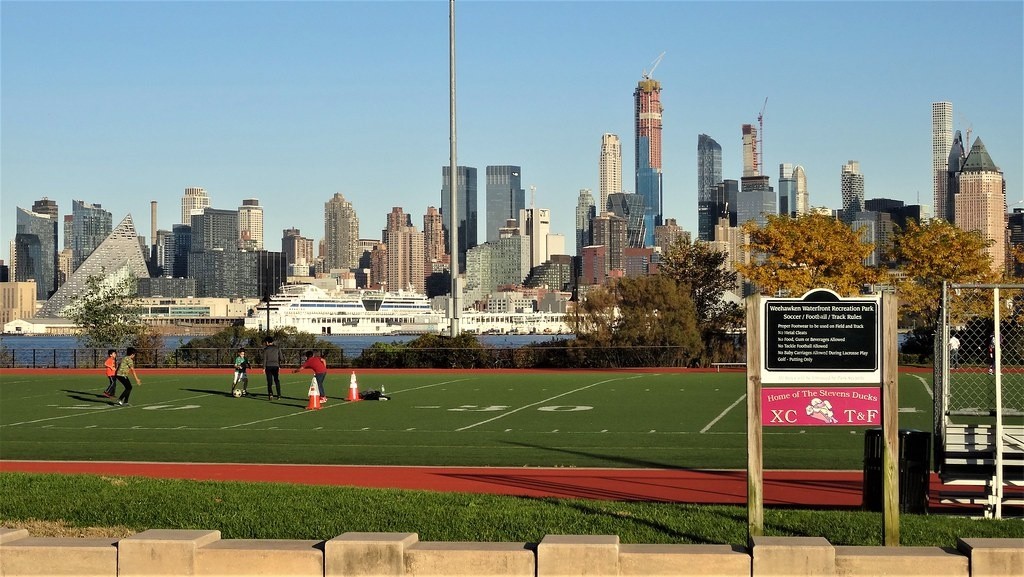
[860,428,933,515]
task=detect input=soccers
[233,389,242,398]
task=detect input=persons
[102,349,119,400]
[292,350,328,404]
[989,329,1003,374]
[949,329,961,370]
[263,336,284,400]
[232,348,253,397]
[117,347,142,407]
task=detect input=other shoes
[278,396,281,400]
[123,402,132,407]
[269,395,274,400]
[243,392,251,396]
[116,399,123,406]
[321,396,328,402]
[103,392,116,399]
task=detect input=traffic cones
[343,370,361,402]
[305,377,324,410]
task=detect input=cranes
[531,177,538,207]
[641,52,666,80]
[758,96,769,176]
[966,124,973,154]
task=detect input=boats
[243,278,448,335]
